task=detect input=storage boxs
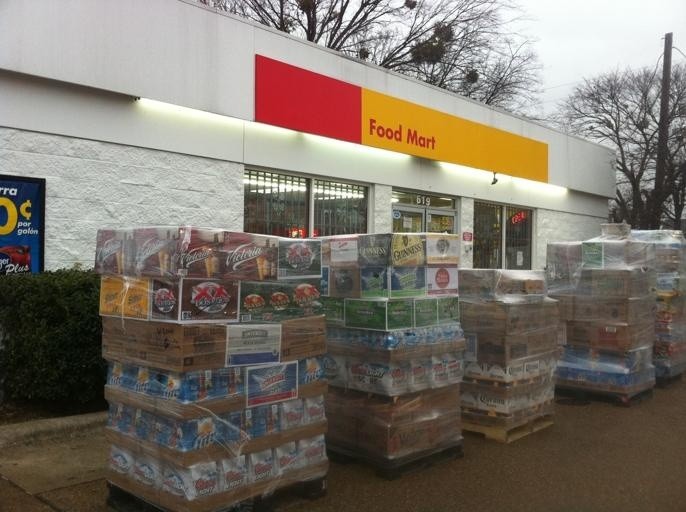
[97,227,330,506]
[316,233,463,475]
[543,229,684,401]
[455,268,560,443]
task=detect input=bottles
[116,229,277,281]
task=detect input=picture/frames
[0,173,46,274]
